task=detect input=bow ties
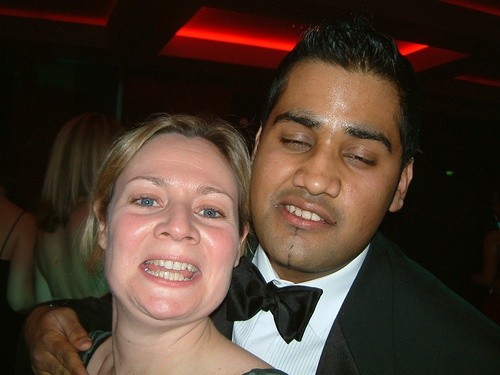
[224,252,325,342]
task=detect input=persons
[35,113,129,306]
[0,17,499,374]
[80,114,289,375]
[482,190,500,325]
[0,186,39,313]
